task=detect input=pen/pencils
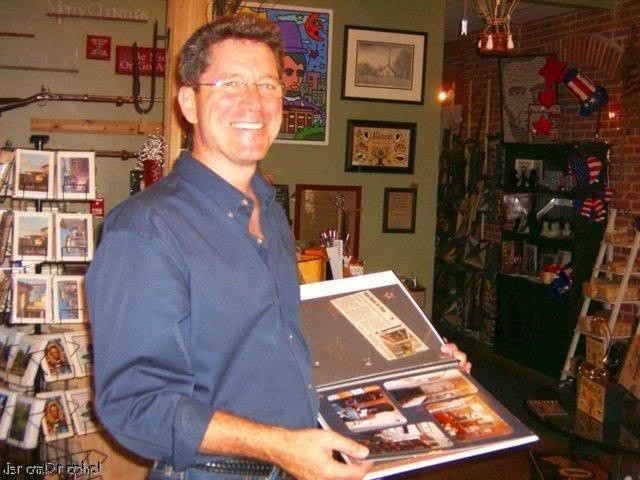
[320,228,350,249]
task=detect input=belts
[197,461,282,477]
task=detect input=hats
[275,21,308,54]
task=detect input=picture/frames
[292,183,365,262]
[340,24,429,106]
[1,143,109,450]
[382,186,418,235]
[343,118,420,174]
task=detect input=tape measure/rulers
[30,118,164,135]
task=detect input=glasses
[193,80,285,101]
[283,69,306,78]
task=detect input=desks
[523,382,640,479]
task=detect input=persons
[83,14,473,480]
[49,346,72,375]
[47,403,68,435]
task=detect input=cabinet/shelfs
[494,140,613,379]
[559,209,640,387]
[232,2,334,149]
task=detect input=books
[300,269,541,480]
